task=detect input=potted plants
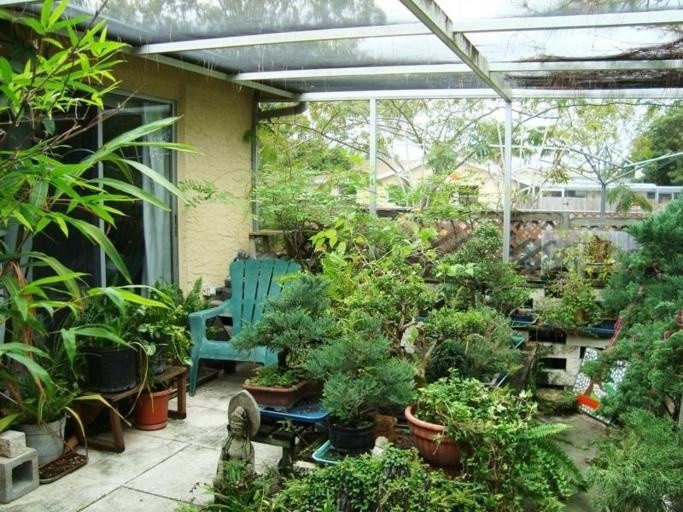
[248,259,497,475]
[0,278,195,477]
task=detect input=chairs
[187,254,301,399]
